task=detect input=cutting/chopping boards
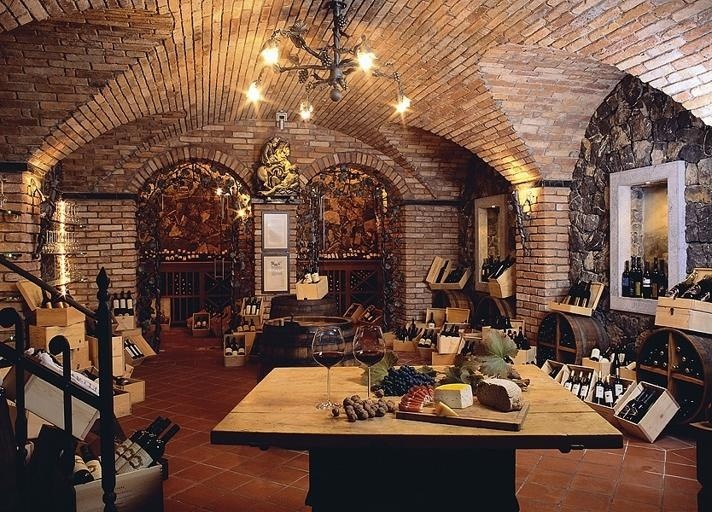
[396,387,532,429]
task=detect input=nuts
[331,389,396,422]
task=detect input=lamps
[242,0,416,129]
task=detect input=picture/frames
[260,252,292,294]
[259,209,292,251]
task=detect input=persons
[257,138,299,197]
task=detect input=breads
[434,383,474,409]
[475,378,524,411]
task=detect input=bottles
[395,307,531,360]
[346,296,386,324]
[620,253,710,301]
[314,246,384,260]
[191,293,259,358]
[37,287,76,310]
[15,415,182,486]
[558,274,593,309]
[301,273,322,284]
[435,255,518,289]
[124,338,152,361]
[109,289,137,318]
[132,247,231,262]
[546,341,658,428]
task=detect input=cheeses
[436,401,458,415]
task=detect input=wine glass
[40,197,85,288]
[308,325,344,410]
[351,321,386,401]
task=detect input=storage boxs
[0,274,170,511]
[185,295,266,367]
[293,273,329,302]
[343,254,712,446]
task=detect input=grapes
[371,365,437,396]
